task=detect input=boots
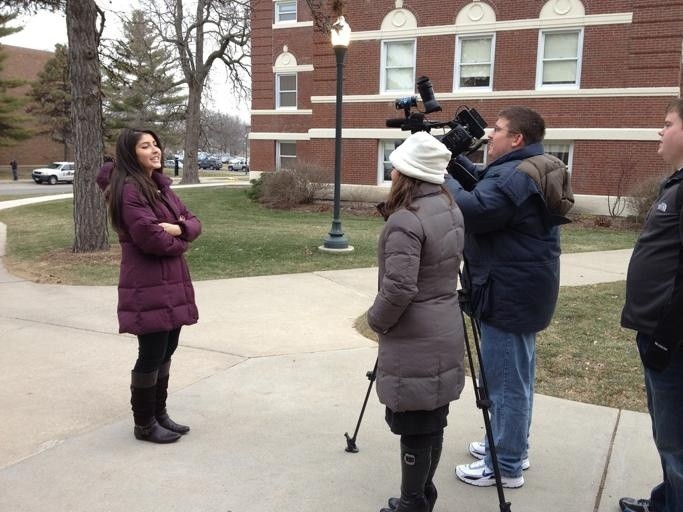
[31,162,76,185]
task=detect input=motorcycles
[344,250,512,511]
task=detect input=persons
[441,105,574,489]
[368,130,466,512]
[97,129,201,442]
[616,100,683,512]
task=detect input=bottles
[388,130,452,185]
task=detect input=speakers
[379,443,442,511]
[131,359,190,443]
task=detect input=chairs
[620,496,650,512]
[469,441,530,470]
[455,457,524,487]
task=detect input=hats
[161,150,250,172]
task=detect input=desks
[317,15,357,257]
